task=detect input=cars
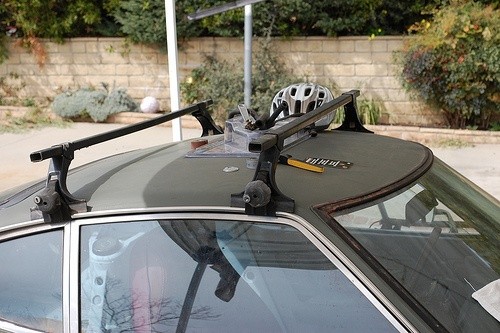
[0,89,499,333]
